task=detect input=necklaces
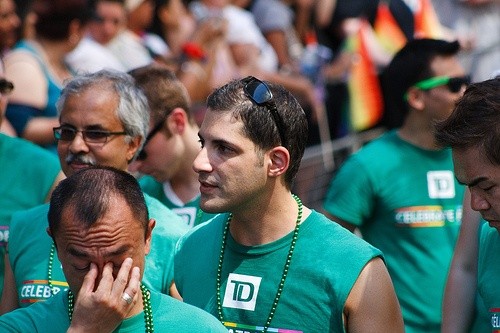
[216,193,302,333]
[68,283,154,333]
[48,239,55,296]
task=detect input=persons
[0,0,500,304]
[321,38,466,333]
[434,76,500,231]
[442,187,500,333]
[173,75,405,333]
[1,69,190,316]
[0,166,230,333]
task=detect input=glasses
[52,126,130,143]
[239,75,287,150]
[135,110,171,162]
[0,80,14,94]
[403,73,472,101]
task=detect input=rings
[122,293,132,305]
